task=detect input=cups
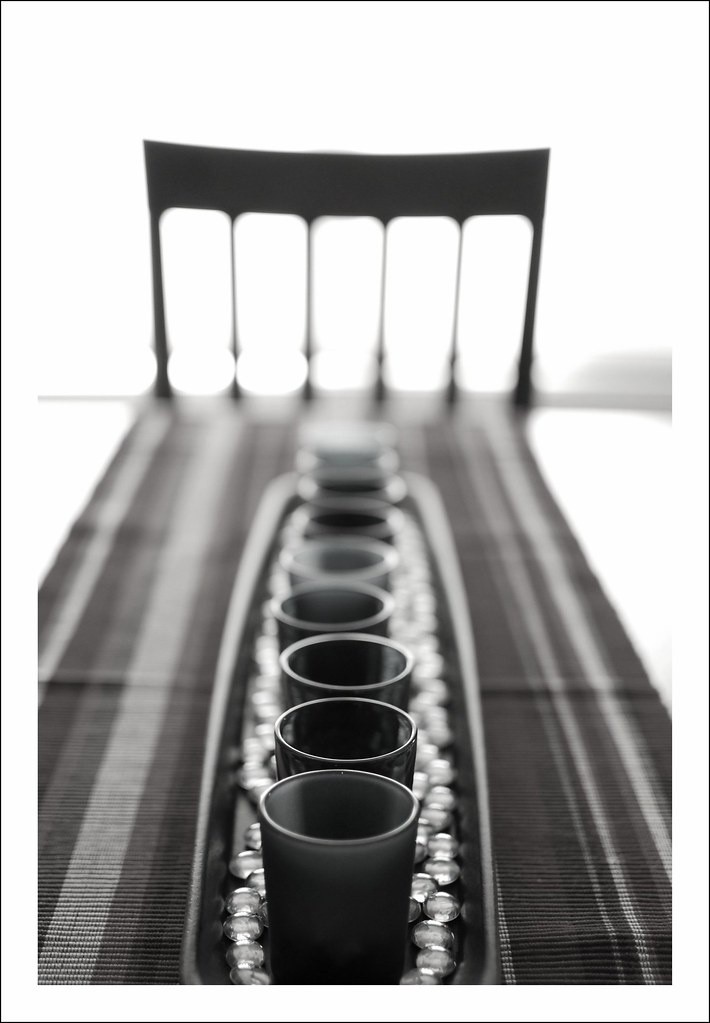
[278,632,415,713]
[272,697,417,793]
[257,767,422,986]
[268,418,409,655]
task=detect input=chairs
[143,140,549,403]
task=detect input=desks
[32,392,671,982]
